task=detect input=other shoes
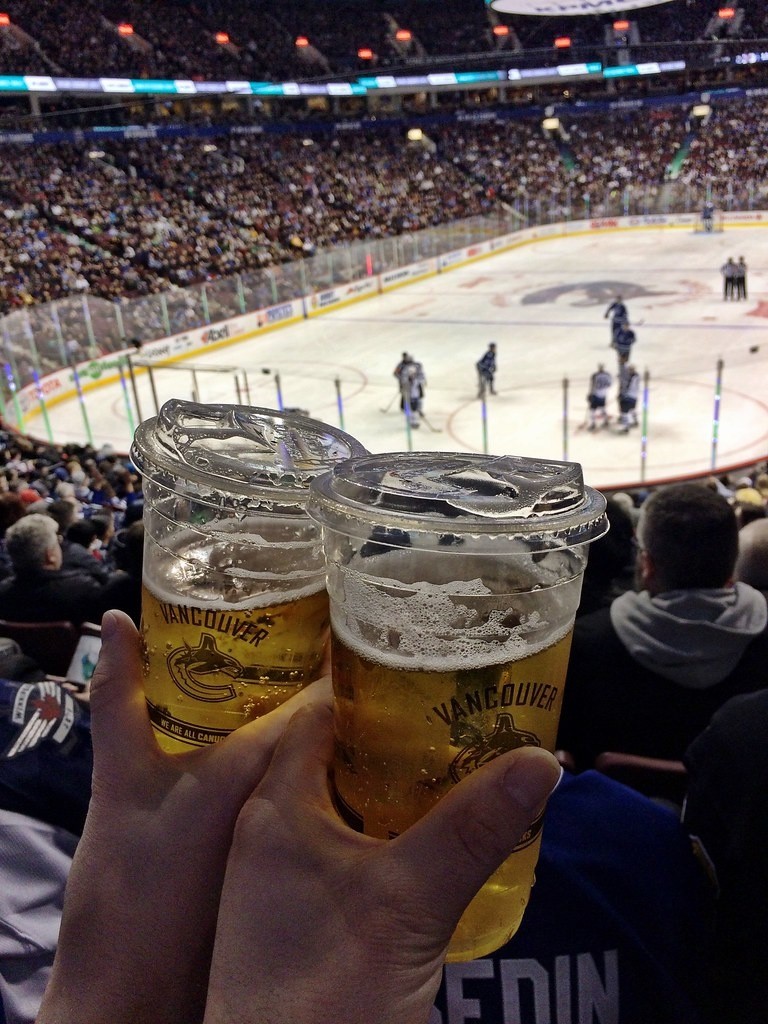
[490,389,496,395]
[600,422,608,428]
[588,424,595,431]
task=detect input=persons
[617,366,638,433]
[0,428,768,1024]
[477,343,496,396]
[587,363,611,430]
[396,352,424,414]
[0,0,768,371]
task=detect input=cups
[309,453,608,966]
[129,399,381,754]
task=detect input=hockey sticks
[380,389,401,414]
[418,408,444,434]
[577,406,592,431]
